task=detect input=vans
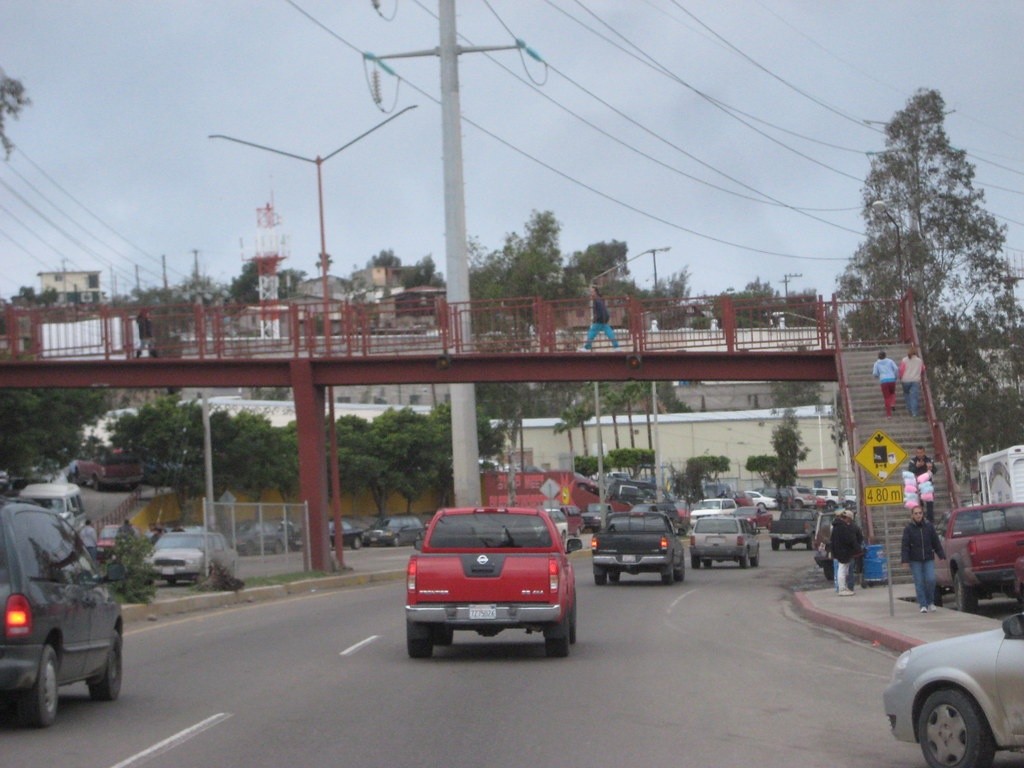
[73,442,147,493]
[689,513,762,569]
[17,481,88,532]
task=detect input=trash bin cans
[862,544,887,582]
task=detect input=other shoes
[891,407,896,411]
[577,347,592,352]
[611,348,620,353]
[839,589,854,596]
[930,604,937,612]
[921,607,927,613]
[887,415,892,419]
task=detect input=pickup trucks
[404,505,584,660]
[590,510,687,585]
[930,499,1024,614]
[768,507,826,551]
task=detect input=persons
[579,286,622,352]
[136,308,159,358]
[872,351,898,419]
[901,506,946,613]
[831,509,864,595]
[899,347,925,417]
[908,446,938,522]
[79,520,97,563]
[115,519,185,546]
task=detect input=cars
[499,482,858,558]
[141,530,240,586]
[95,511,427,587]
[879,612,1024,768]
[0,495,127,730]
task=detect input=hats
[842,510,854,520]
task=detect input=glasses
[914,512,922,515]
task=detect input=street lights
[647,246,673,330]
[871,200,907,344]
